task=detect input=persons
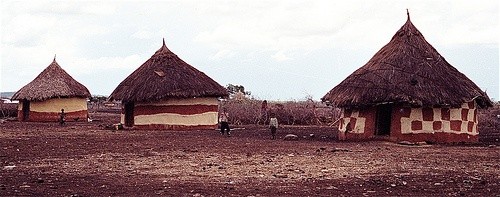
[218,107,232,135]
[268,113,279,140]
[59,109,68,126]
